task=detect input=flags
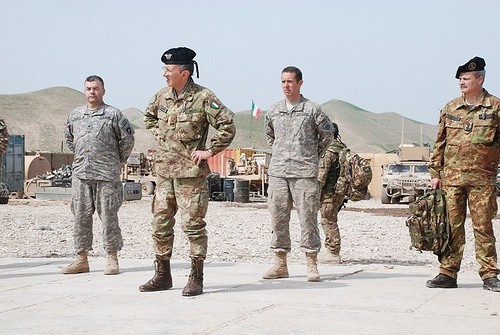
[251,102,262,120]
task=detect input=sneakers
[483,278,500,292]
[426,274,457,288]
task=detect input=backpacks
[332,143,372,201]
[407,189,450,255]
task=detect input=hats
[456,56,486,78]
[331,122,339,139]
[161,47,197,64]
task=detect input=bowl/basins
[0,196,9,204]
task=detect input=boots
[139,260,172,291]
[60,251,89,274]
[306,252,320,282]
[318,249,340,263]
[104,252,119,274]
[182,259,204,295]
[262,253,289,278]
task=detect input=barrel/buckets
[235,180,249,202]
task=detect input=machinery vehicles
[380,162,433,203]
[119,153,165,195]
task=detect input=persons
[139,48,237,296]
[425,56,500,292]
[61,75,136,275]
[262,66,336,282]
[319,121,346,265]
